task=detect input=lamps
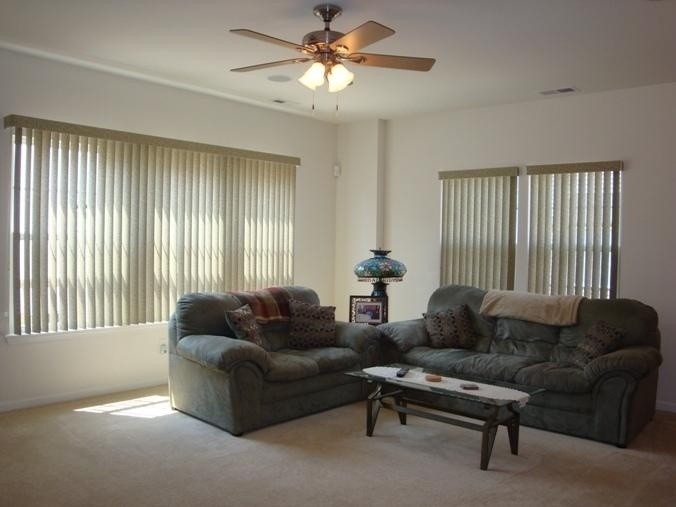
[352,247,407,297]
[299,53,354,93]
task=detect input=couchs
[376,285,663,449]
[167,287,380,437]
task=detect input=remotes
[396,367,409,377]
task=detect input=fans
[228,4,436,73]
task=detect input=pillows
[422,304,474,349]
[225,298,336,352]
[569,318,627,371]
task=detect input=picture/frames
[349,295,388,324]
